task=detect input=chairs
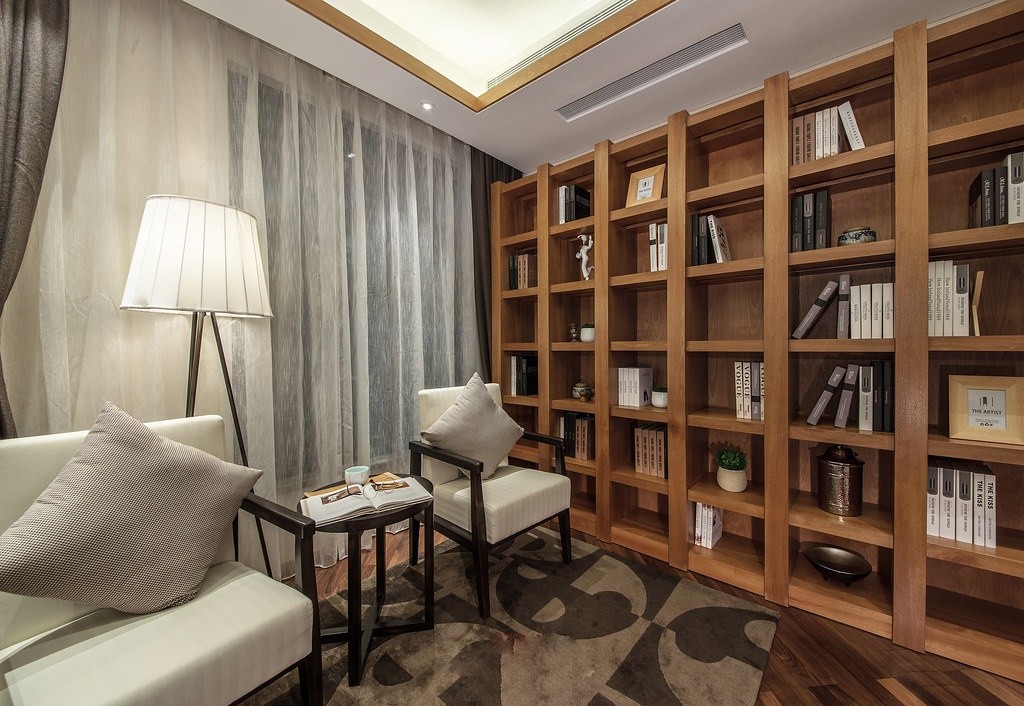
[408,382,572,620]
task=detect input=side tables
[296,473,434,687]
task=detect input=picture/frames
[948,375,1024,446]
[626,163,665,208]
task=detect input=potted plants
[714,449,749,493]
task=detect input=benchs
[0,414,324,706]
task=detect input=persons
[576,235,595,280]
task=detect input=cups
[345,466,369,485]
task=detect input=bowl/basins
[573,383,590,398]
[838,226,877,247]
[807,547,872,587]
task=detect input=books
[646,223,667,270]
[618,367,653,407]
[695,502,722,549]
[299,477,435,528]
[510,355,538,396]
[734,361,764,420]
[559,411,594,460]
[968,166,1008,229]
[559,185,590,224]
[927,458,996,548]
[692,214,732,266]
[508,254,537,290]
[793,100,866,166]
[635,424,666,479]
[792,190,832,253]
[1002,152,1024,225]
[792,274,893,339]
[928,260,970,336]
[807,359,891,431]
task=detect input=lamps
[117,193,275,581]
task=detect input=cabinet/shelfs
[491,8,1024,687]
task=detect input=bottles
[818,445,865,517]
[568,324,580,342]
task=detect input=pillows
[419,372,525,481]
[0,401,262,615]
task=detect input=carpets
[233,526,779,706]
